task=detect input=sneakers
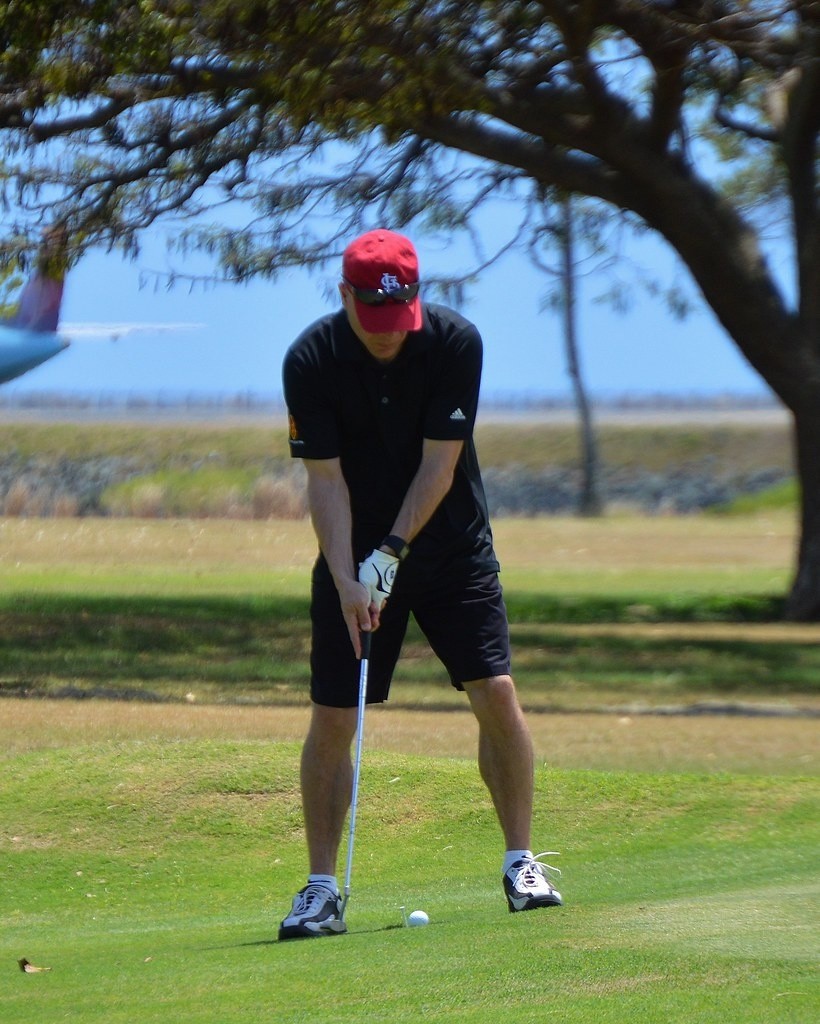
[279,885,347,940]
[503,851,563,912]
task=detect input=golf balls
[408,910,429,926]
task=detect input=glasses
[341,273,420,308]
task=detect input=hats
[341,229,423,334]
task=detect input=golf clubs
[318,631,372,934]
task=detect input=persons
[278,228,563,939]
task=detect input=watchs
[382,535,410,560]
